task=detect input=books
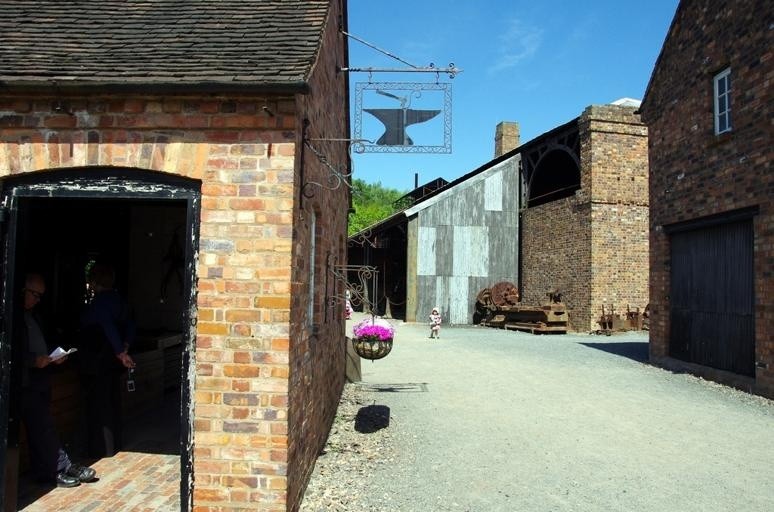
[48,347,78,362]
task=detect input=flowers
[353,316,395,340]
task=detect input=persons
[75,260,137,458]
[20,275,96,488]
[428,307,442,338]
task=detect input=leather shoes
[67,464,95,481]
[38,472,80,486]
